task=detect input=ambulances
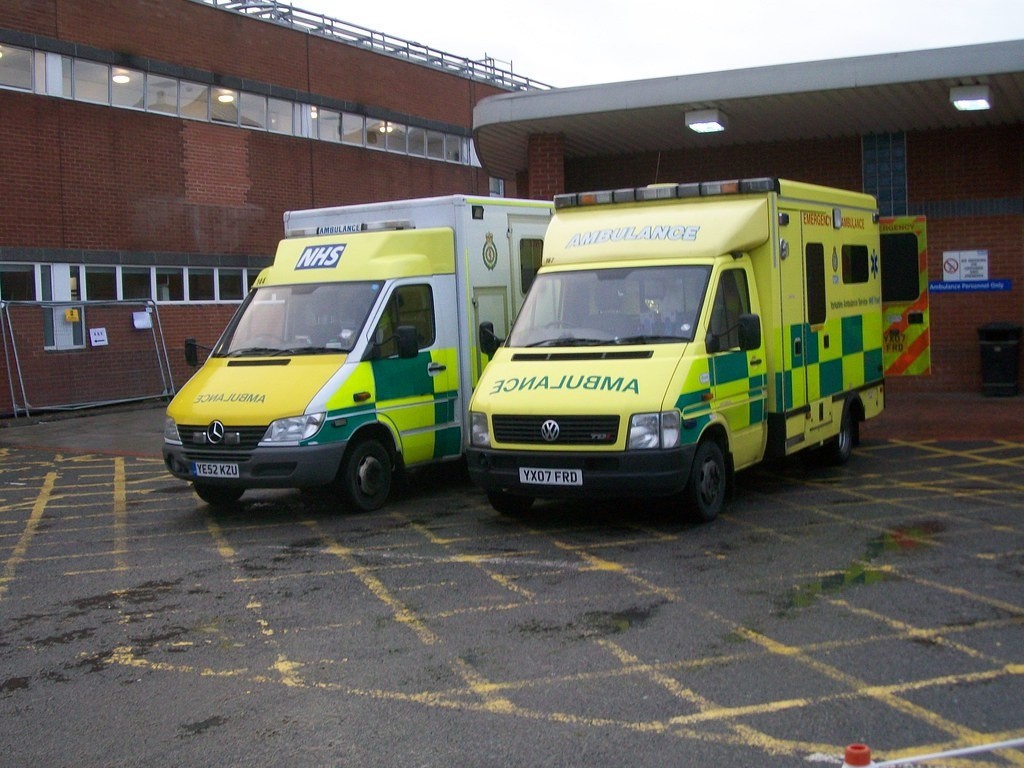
[464,178,934,526]
[162,194,642,516]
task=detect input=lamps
[217,88,234,102]
[112,67,130,84]
[949,83,995,111]
[311,106,317,119]
[379,119,393,133]
[684,109,730,133]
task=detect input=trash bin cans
[978,322,1024,396]
